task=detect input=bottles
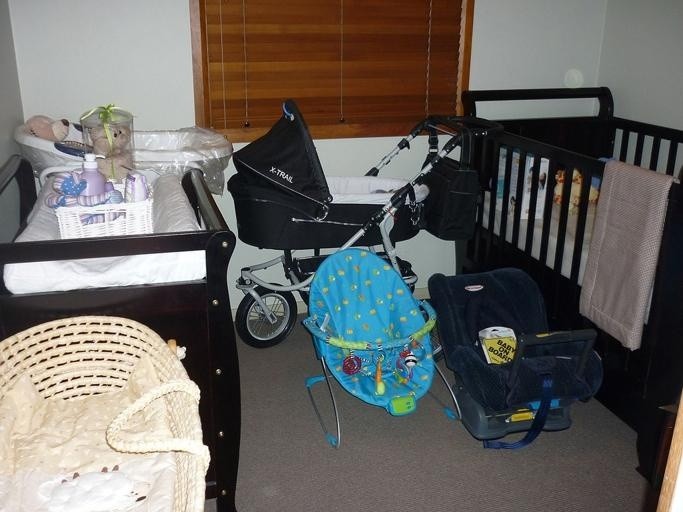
[123,174,148,204]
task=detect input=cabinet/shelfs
[1,155,241,512]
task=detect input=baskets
[0,316,211,512]
[56,183,153,240]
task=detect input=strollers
[226,98,498,349]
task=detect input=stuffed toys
[25,114,94,157]
[91,125,134,180]
[45,169,148,226]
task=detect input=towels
[580,159,680,351]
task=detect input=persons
[508,196,516,218]
[527,167,546,191]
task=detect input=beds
[463,86,683,432]
